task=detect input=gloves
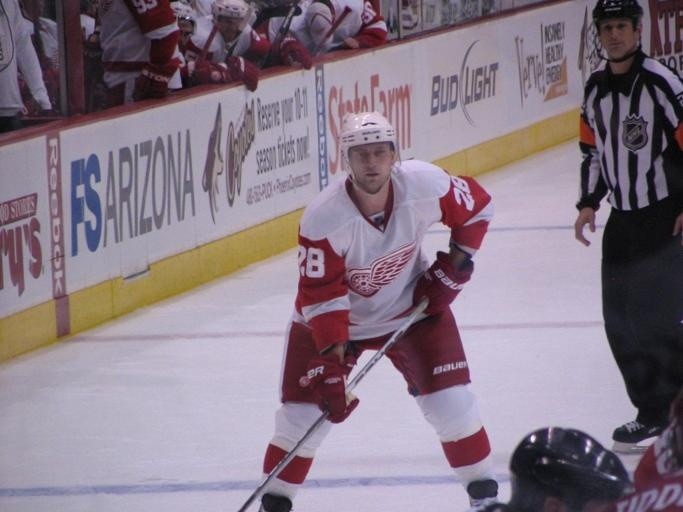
[133,55,178,102]
[413,250,472,317]
[187,55,230,83]
[227,48,262,93]
[276,35,314,73]
[303,352,361,426]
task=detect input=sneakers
[612,417,666,444]
[465,479,507,511]
[256,492,292,512]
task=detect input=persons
[481,427,629,512]
[260,112,499,512]
[574,1,683,451]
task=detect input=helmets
[338,111,402,185]
[211,0,249,27]
[592,1,644,28]
[508,426,630,500]
[170,1,199,28]
